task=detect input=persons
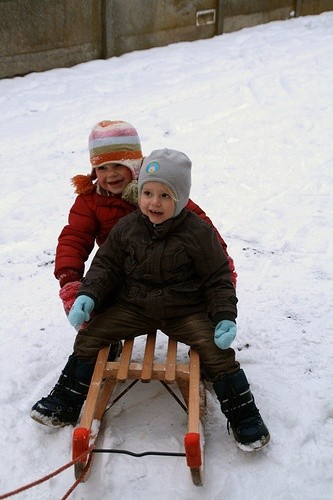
[54,120,239,361]
[31,148,271,451]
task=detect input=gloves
[214,320,237,350]
[227,255,238,290]
[59,281,94,330]
[67,294,95,327]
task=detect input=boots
[213,368,271,453]
[30,352,94,428]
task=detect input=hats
[71,120,143,203]
[138,148,192,217]
